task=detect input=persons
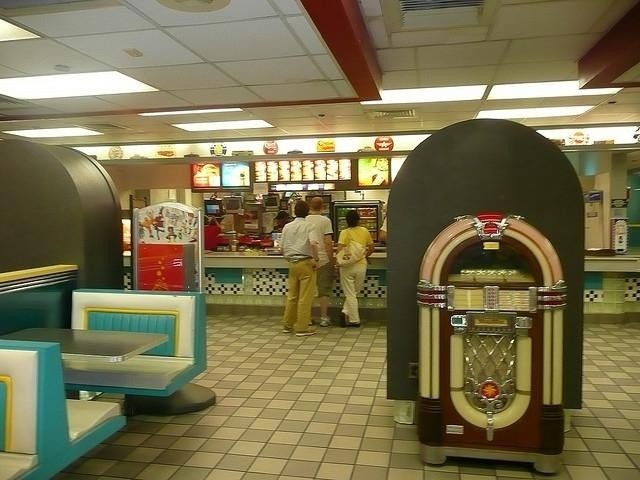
[203,214,221,251]
[279,200,320,336]
[336,209,375,328]
[306,197,338,327]
[273,211,295,231]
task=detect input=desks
[2,326,168,362]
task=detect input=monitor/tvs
[205,200,222,216]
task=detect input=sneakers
[320,317,336,326]
[280,315,316,337]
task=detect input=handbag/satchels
[336,229,368,265]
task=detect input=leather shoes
[345,323,360,328]
[337,313,348,327]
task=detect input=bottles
[231,234,237,252]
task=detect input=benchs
[1,338,135,479]
[64,288,217,415]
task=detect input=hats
[274,211,288,220]
